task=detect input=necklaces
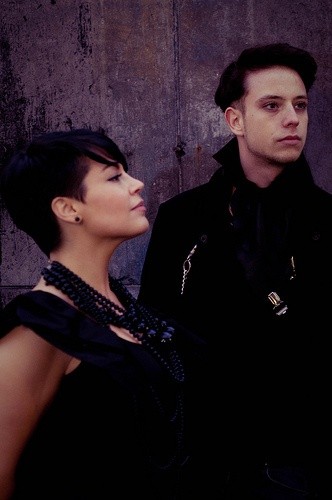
[42,262,185,383]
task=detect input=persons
[138,44,332,500]
[0,129,210,500]
